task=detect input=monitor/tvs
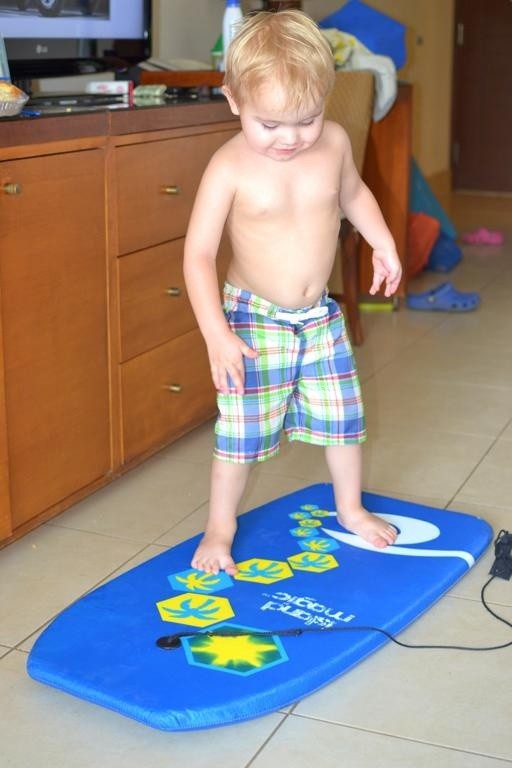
[0,0,151,79]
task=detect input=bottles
[222,0,243,73]
[209,33,224,100]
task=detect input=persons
[179,5,407,580]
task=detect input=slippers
[402,280,484,316]
[460,224,506,250]
